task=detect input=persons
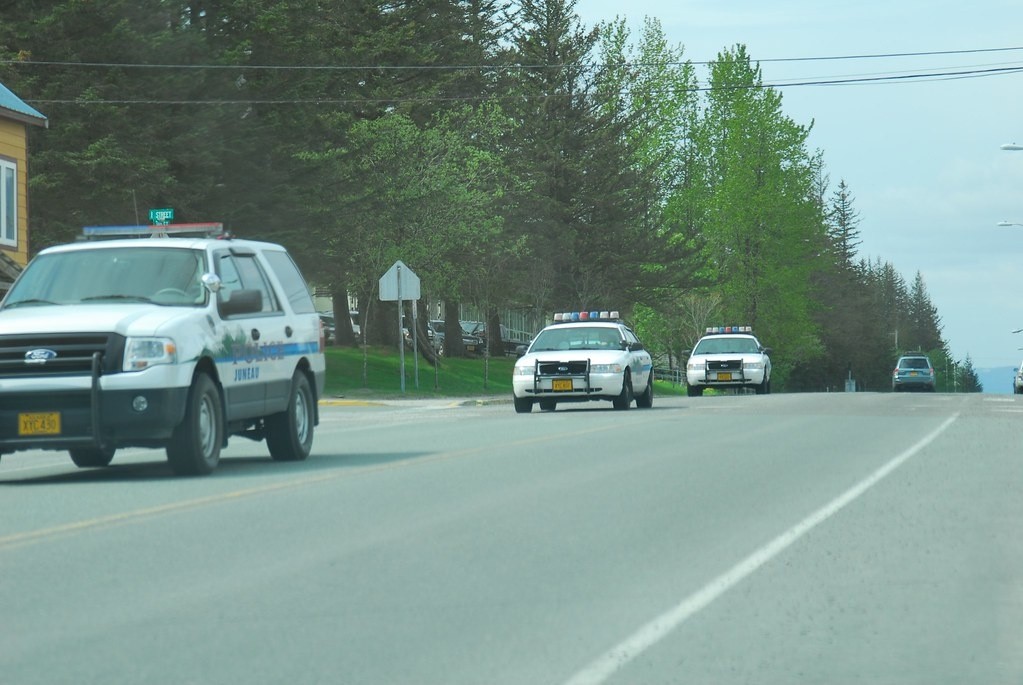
[738,341,754,352]
[594,330,617,349]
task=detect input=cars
[1013,361,1023,394]
[316,310,362,343]
[892,351,938,393]
[402,314,441,354]
[431,319,485,355]
[510,310,656,409]
[685,326,775,395]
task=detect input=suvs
[1,223,328,468]
[458,320,509,343]
